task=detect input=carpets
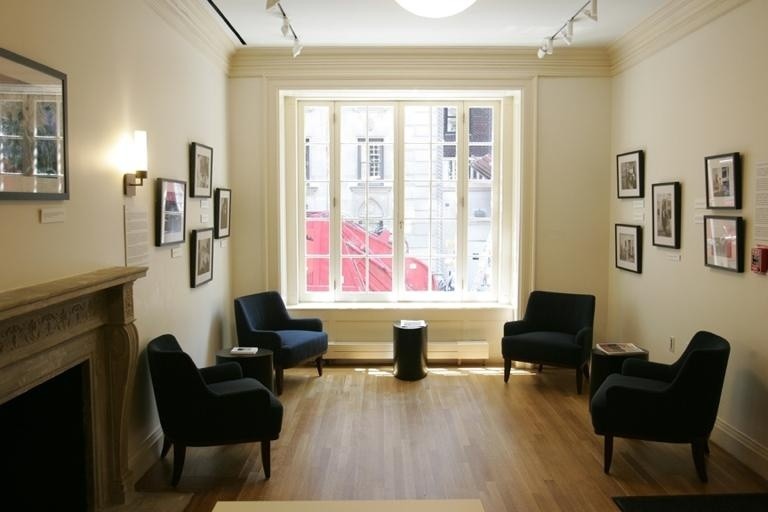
[611,493,768,511]
[210,498,489,512]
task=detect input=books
[596,342,645,354]
[400,319,426,327]
[231,345,259,355]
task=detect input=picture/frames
[188,140,215,199]
[614,223,644,274]
[704,151,743,210]
[651,181,683,249]
[212,186,232,240]
[155,177,187,247]
[615,150,646,198]
[189,226,214,289]
[703,214,746,274]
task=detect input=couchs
[591,326,730,483]
[146,332,288,488]
[232,290,331,397]
[498,289,598,395]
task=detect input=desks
[589,343,650,411]
[211,345,275,393]
[390,319,430,382]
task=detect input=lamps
[123,129,150,198]
[538,1,598,61]
[264,0,305,60]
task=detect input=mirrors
[0,47,71,204]
